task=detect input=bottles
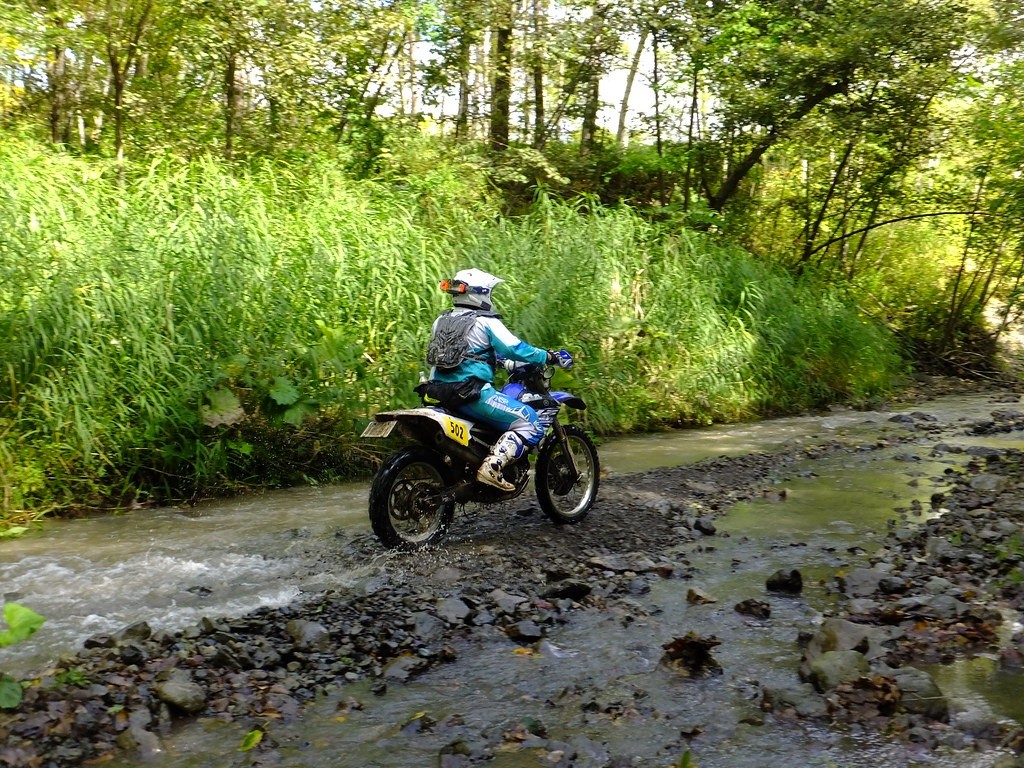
[419,370,428,385]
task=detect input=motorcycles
[360,349,600,555]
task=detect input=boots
[476,430,528,491]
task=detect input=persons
[412,268,558,492]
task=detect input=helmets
[452,268,505,310]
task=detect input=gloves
[546,350,560,365]
[496,354,506,368]
